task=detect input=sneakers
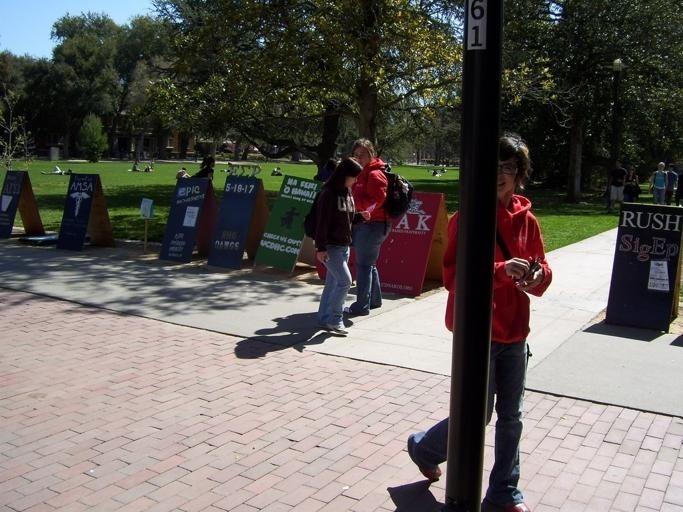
[344,307,353,314]
[326,320,349,335]
[422,466,442,481]
[501,499,530,512]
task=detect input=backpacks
[303,191,323,241]
[380,163,414,218]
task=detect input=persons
[342,135,395,317]
[39,165,74,176]
[663,163,679,205]
[609,160,628,210]
[650,161,668,204]
[430,166,447,177]
[317,160,338,183]
[189,156,216,183]
[131,159,154,172]
[0,159,12,169]
[269,167,283,176]
[176,167,192,180]
[314,159,373,335]
[406,131,554,512]
[675,170,683,206]
[623,166,639,203]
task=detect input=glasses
[498,162,519,176]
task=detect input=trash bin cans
[50,147,60,161]
